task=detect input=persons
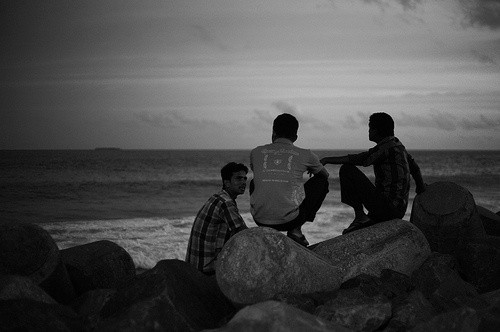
[250,113,329,248]
[185,162,249,276]
[307,113,429,235]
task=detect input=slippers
[343,219,372,235]
[287,232,309,247]
